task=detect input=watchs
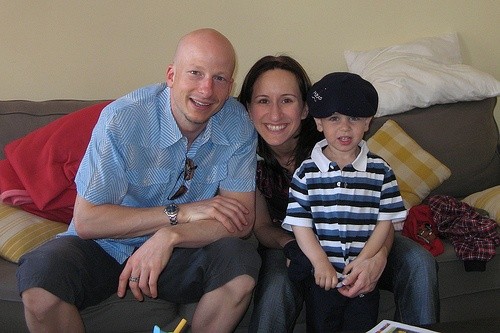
[163,203,180,225]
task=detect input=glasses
[168,155,198,200]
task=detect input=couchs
[0,95,500,333]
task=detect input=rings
[129,277,139,282]
[359,293,365,297]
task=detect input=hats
[402,205,445,256]
[309,72,378,118]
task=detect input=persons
[281,73,407,333]
[16,28,262,333]
[237,51,438,333]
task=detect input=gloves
[285,239,313,286]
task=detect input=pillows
[345,32,500,117]
[0,198,69,265]
[461,186,500,225]
[365,119,451,211]
